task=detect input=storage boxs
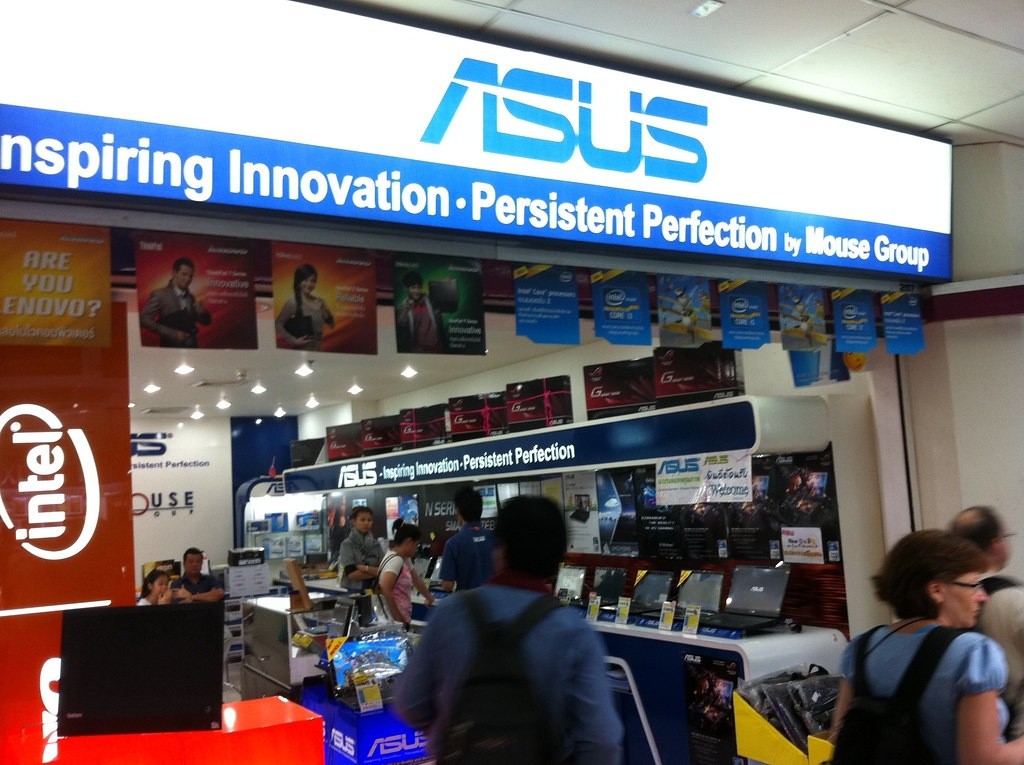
[327,338,739,465]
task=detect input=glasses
[995,530,1017,541]
[942,575,989,592]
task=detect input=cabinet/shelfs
[224,586,244,662]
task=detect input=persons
[137,570,193,607]
[438,486,494,593]
[139,257,211,349]
[827,529,1024,765]
[370,518,432,632]
[396,270,443,353]
[388,495,624,765]
[947,507,1024,743]
[170,548,224,602]
[275,264,334,350]
[338,506,385,592]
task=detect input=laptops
[554,565,792,632]
[413,557,456,601]
[325,627,416,714]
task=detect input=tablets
[57,600,225,738]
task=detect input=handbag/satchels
[371,553,405,624]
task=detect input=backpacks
[433,587,566,765]
[821,626,970,764]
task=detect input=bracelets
[366,566,368,572]
